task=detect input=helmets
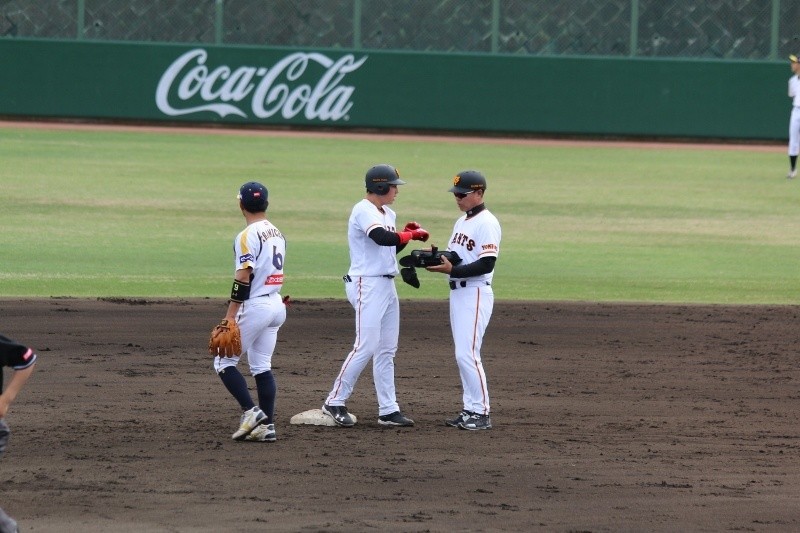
[365,165,407,194]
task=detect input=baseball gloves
[208,317,242,359]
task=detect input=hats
[447,171,486,194]
[237,182,269,202]
[788,54,800,62]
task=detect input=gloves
[402,222,420,243]
[397,228,430,246]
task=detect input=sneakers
[458,413,492,430]
[240,424,276,441]
[231,406,269,439]
[446,411,473,426]
[322,403,354,426]
[377,411,414,426]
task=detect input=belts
[382,274,395,278]
[449,280,489,289]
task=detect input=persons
[0,334,37,533]
[426,171,502,431]
[322,164,429,427]
[786,52,800,178]
[208,181,287,442]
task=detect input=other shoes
[788,168,797,176]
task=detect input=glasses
[454,190,477,198]
